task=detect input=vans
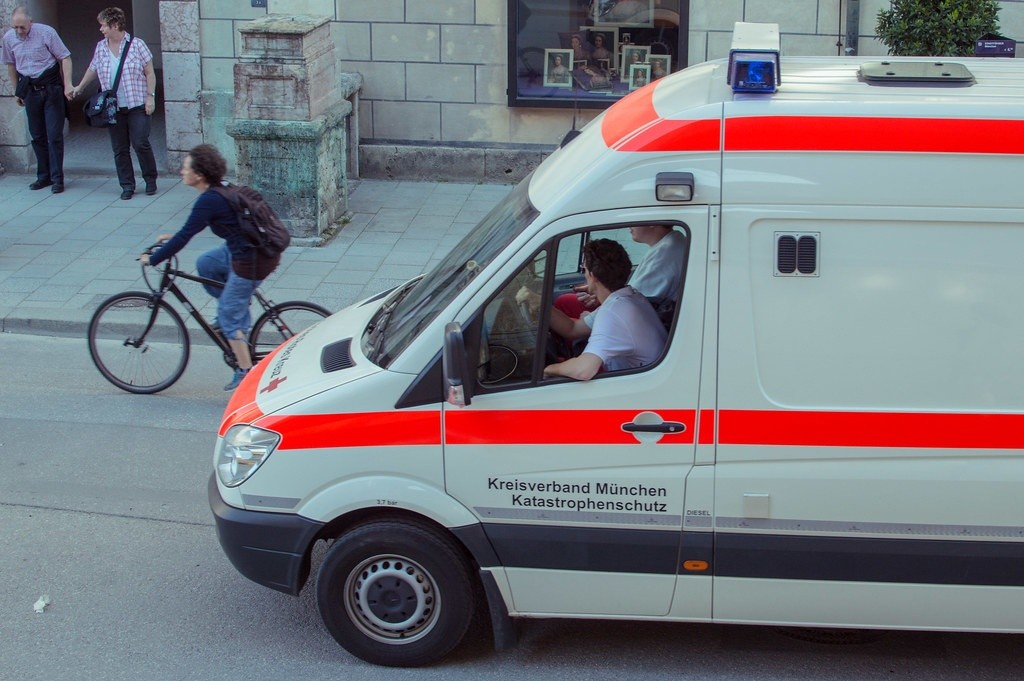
[205,20,1024,670]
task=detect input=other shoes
[52,182,64,193]
[30,180,51,189]
[146,181,157,194]
[121,190,134,199]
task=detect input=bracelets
[147,92,155,96]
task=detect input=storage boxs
[975,33,1016,57]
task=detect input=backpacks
[206,183,290,258]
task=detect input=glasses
[579,264,592,274]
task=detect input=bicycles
[85,240,332,395]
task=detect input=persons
[515,238,669,380]
[138,145,281,392]
[553,225,686,348]
[1,4,75,194]
[72,7,156,199]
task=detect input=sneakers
[208,318,220,329]
[224,368,250,392]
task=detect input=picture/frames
[543,0,671,91]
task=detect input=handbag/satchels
[83,90,117,127]
